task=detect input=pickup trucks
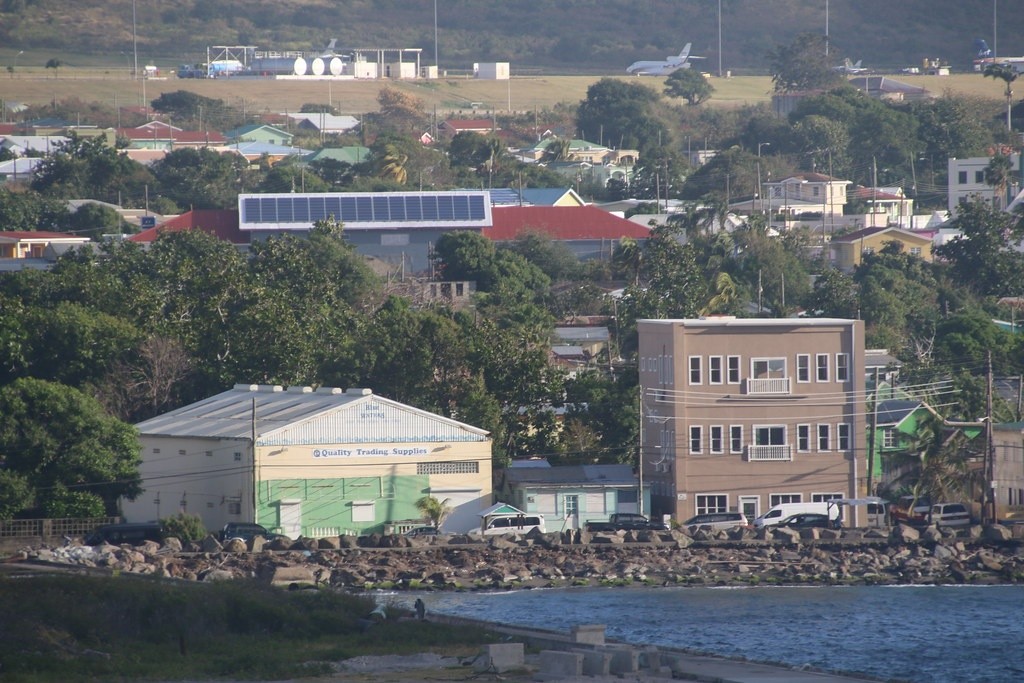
[583,513,669,533]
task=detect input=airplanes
[625,42,706,77]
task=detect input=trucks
[177,60,242,78]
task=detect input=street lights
[758,142,770,207]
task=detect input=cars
[775,513,829,530]
[218,521,289,545]
[402,527,442,535]
[892,496,971,527]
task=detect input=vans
[681,512,749,534]
[753,502,841,531]
[468,513,546,538]
[82,522,165,546]
